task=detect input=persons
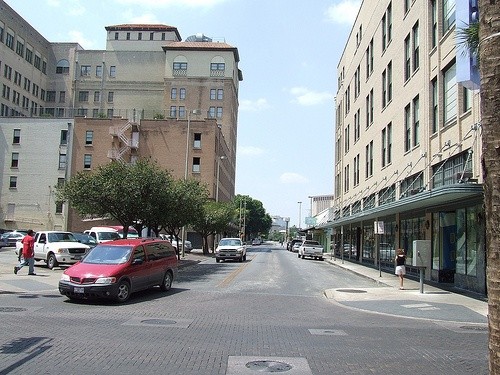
[14,229,37,275]
[393,249,408,290]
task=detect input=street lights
[215,156,227,248]
[181,109,202,259]
[298,201,302,228]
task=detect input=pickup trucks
[14,231,90,270]
[378,242,395,260]
[215,238,247,263]
[298,240,324,260]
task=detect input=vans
[58,237,178,305]
[71,225,139,249]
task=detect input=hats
[27,230,36,235]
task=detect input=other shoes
[14,267,17,274]
[28,273,36,275]
[399,287,404,290]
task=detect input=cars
[252,238,263,246]
[343,243,371,258]
[0,232,27,248]
[287,239,303,253]
[159,233,192,255]
